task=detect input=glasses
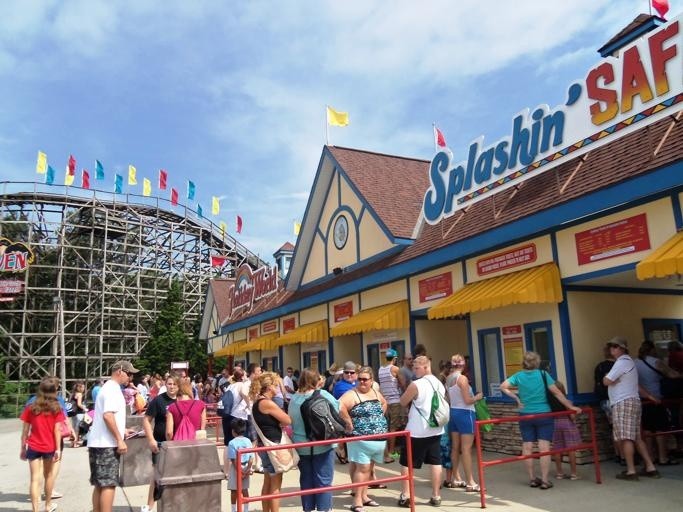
[287,370,294,374]
[343,370,357,374]
[358,376,373,382]
[404,357,416,360]
[222,373,226,376]
[122,369,134,377]
[211,379,214,383]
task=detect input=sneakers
[139,504,156,512]
[384,456,395,463]
[43,491,63,499]
[619,459,639,466]
[639,467,661,478]
[439,483,442,490]
[615,470,640,481]
[615,456,620,463]
[430,496,441,506]
[37,495,43,503]
[398,497,412,508]
[387,450,402,459]
[443,480,452,488]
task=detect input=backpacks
[217,382,241,416]
[67,399,77,417]
[173,400,196,440]
[299,389,346,440]
[412,377,449,427]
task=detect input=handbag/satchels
[539,367,569,413]
[448,373,460,393]
[84,409,92,424]
[251,406,301,474]
[61,413,71,438]
[640,356,670,377]
[473,397,495,433]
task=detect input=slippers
[350,505,364,512]
[369,483,388,489]
[363,499,380,507]
[346,459,349,463]
[652,458,659,464]
[336,450,346,464]
[658,457,679,465]
[254,466,265,473]
[46,503,58,512]
[350,492,356,496]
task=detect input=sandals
[555,473,569,479]
[569,474,581,480]
[465,484,487,491]
[452,480,467,487]
[529,477,541,487]
[540,481,554,489]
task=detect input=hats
[606,336,630,350]
[329,363,337,375]
[335,368,344,375]
[111,360,141,374]
[385,348,401,358]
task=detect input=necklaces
[261,392,268,398]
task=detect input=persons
[338,366,388,512]
[141,374,180,512]
[284,367,296,403]
[594,343,661,467]
[26,376,77,500]
[666,340,683,464]
[123,372,170,414]
[439,359,453,488]
[500,352,582,489]
[20,378,66,512]
[92,378,104,409]
[446,353,486,492]
[413,343,427,358]
[634,341,683,467]
[166,381,206,442]
[224,369,250,479]
[602,337,659,481]
[539,360,582,481]
[246,363,264,474]
[333,361,359,400]
[398,356,450,508]
[397,355,417,391]
[69,383,89,448]
[248,371,292,512]
[218,369,232,387]
[191,372,221,403]
[170,369,187,382]
[227,418,255,512]
[87,361,140,512]
[272,369,288,413]
[288,367,353,512]
[378,349,403,459]
